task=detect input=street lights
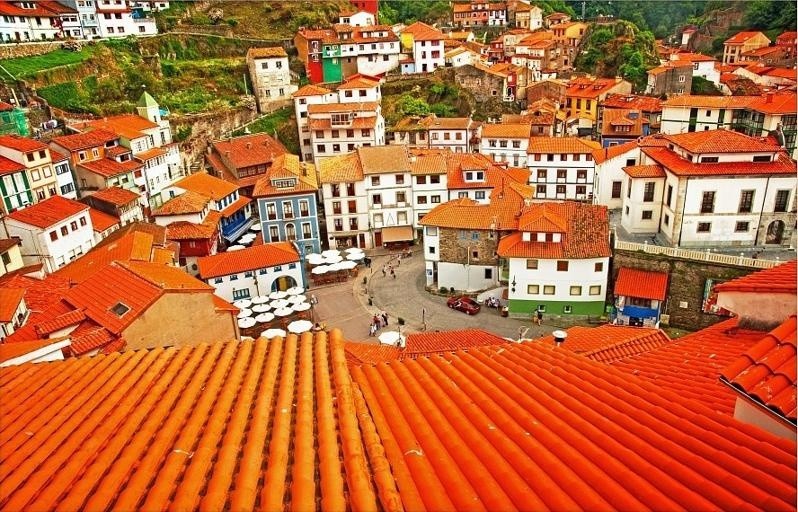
[309,293,320,324]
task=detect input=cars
[445,295,482,316]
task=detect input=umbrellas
[304,246,365,277]
[378,331,402,345]
[233,285,313,341]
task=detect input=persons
[538,313,543,325]
[754,251,760,259]
[364,248,414,278]
[368,312,388,336]
[540,334,544,339]
[534,309,538,317]
[314,323,321,334]
[487,297,500,310]
